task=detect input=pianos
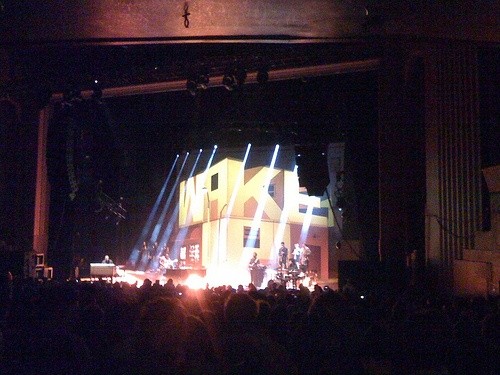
[89,264,121,281]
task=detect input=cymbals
[277,269,288,271]
[288,271,298,274]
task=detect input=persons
[0,242,500,375]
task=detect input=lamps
[199,72,210,89]
[222,74,235,91]
[187,74,199,95]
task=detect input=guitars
[247,256,260,271]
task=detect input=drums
[277,272,282,276]
[297,275,306,280]
[284,275,292,281]
